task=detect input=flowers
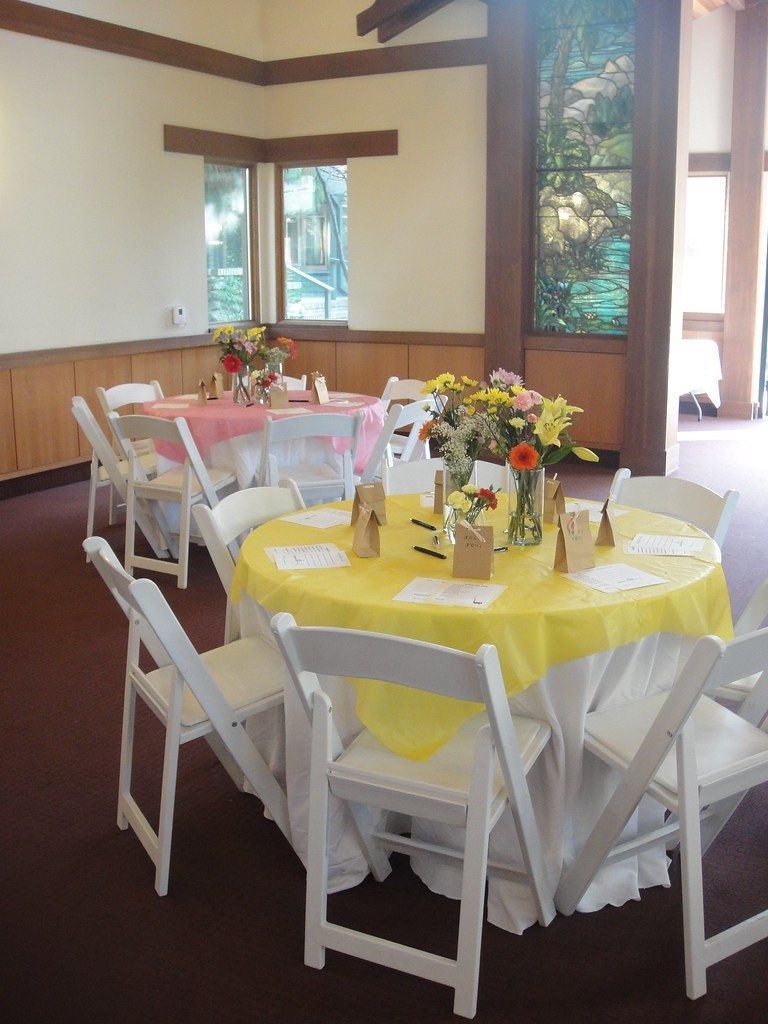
[466,369,599,469]
[418,372,497,462]
[212,324,265,372]
[447,485,497,513]
[249,372,277,387]
[247,326,296,364]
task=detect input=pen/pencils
[412,545,447,559]
[289,400,309,402]
[494,546,509,552]
[244,403,254,408]
[409,518,436,531]
[207,398,218,400]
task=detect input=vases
[446,506,485,544]
[508,467,545,546]
[255,385,270,403]
[232,365,251,403]
[264,362,283,383]
[443,460,477,533]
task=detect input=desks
[226,495,724,935]
[142,391,394,542]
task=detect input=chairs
[68,383,767,1018]
